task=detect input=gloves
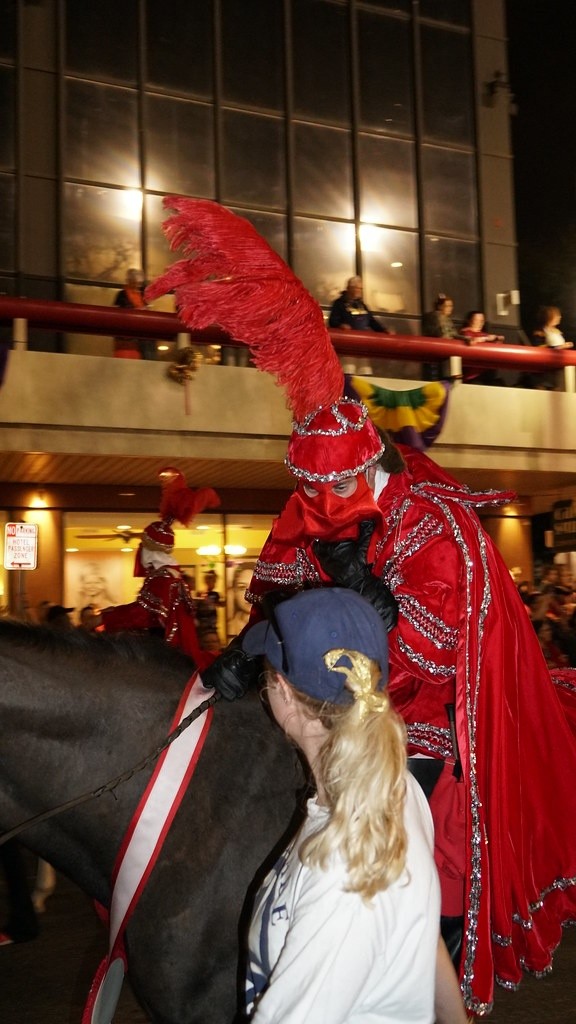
[312,521,399,636]
[202,637,259,701]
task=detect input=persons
[513,303,576,390]
[510,564,576,668]
[142,196,576,1018]
[86,466,225,657]
[241,589,469,1024]
[0,568,219,659]
[111,270,157,360]
[324,274,393,374]
[419,292,509,383]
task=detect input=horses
[1,614,318,1024]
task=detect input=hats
[243,587,389,705]
[142,194,386,483]
[140,467,220,556]
[46,605,75,620]
